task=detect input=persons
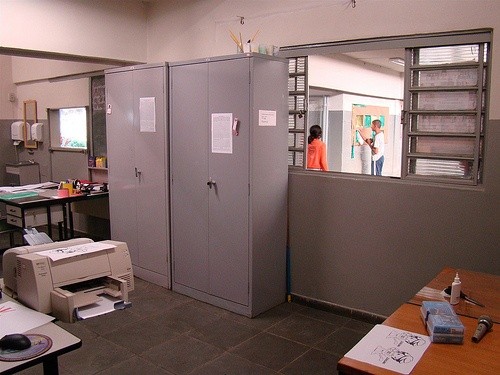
[306,125,328,171]
[357,119,385,176]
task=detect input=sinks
[5,161,40,175]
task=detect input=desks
[337,303,500,375]
[0,292,82,375]
[407,266,500,323]
[0,184,110,247]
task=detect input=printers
[3,228,134,321]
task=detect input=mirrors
[24,100,36,148]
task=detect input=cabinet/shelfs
[169,51,289,318]
[104,61,171,288]
[5,203,64,246]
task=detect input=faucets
[28,159,34,163]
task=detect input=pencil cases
[419,299,465,343]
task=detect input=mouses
[0,334,31,349]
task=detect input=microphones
[472,315,493,341]
